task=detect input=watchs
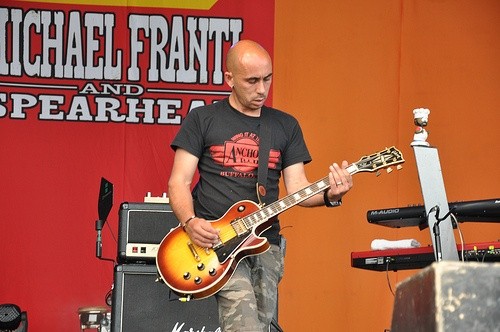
[324,189,342,208]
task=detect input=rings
[337,182,342,185]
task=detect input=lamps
[0,303,28,332]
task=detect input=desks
[390,261,500,332]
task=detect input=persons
[165,40,354,332]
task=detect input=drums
[79,306,107,330]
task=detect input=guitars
[155,146,406,300]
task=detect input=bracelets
[182,216,196,231]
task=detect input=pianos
[367,199,500,229]
[351,241,500,270]
